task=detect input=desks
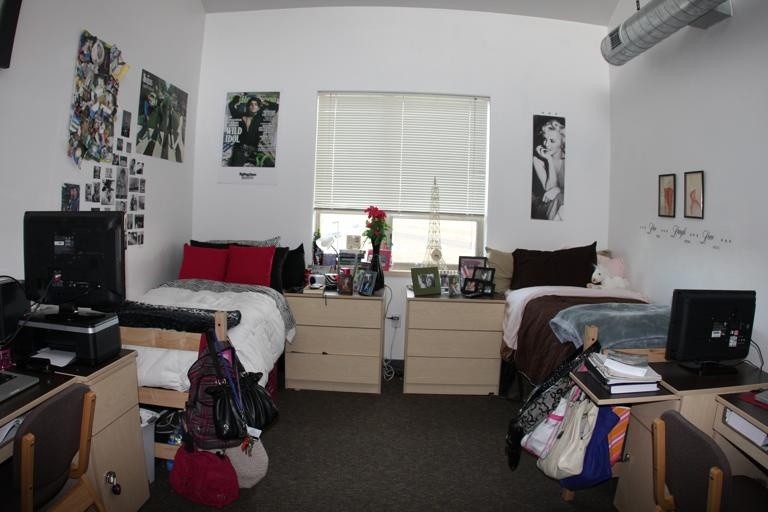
[569,344,768,512]
[0,348,151,511]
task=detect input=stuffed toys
[586,262,630,291]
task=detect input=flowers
[361,204,392,246]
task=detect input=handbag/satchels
[167,330,278,508]
[505,340,631,492]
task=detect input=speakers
[0,0,22,68]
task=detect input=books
[583,347,663,396]
[338,248,367,266]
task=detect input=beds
[118,274,298,461]
[501,277,671,504]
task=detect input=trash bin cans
[138,408,160,485]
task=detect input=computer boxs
[0,278,31,339]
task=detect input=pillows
[178,234,308,294]
[487,241,628,296]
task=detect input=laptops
[0,370,40,401]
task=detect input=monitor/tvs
[23,210,126,319]
[664,288,757,375]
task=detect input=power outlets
[393,314,401,329]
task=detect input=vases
[369,246,385,296]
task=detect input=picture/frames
[351,261,378,296]
[411,256,496,297]
[658,173,676,218]
[684,170,705,219]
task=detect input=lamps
[321,236,339,273]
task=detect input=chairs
[650,408,768,512]
[0,382,107,512]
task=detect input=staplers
[19,357,55,375]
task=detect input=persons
[137,91,186,152]
[340,270,373,293]
[116,156,145,213]
[425,275,434,289]
[531,117,568,223]
[441,258,502,298]
[419,274,428,289]
[226,91,279,167]
[61,183,80,210]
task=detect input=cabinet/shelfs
[283,290,384,396]
[402,287,506,397]
